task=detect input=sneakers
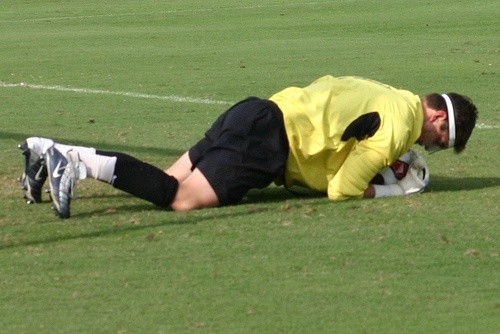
[18,136,54,204]
[39,140,87,218]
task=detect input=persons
[19,75,478,220]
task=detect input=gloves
[373,156,429,198]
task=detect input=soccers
[373,150,430,194]
